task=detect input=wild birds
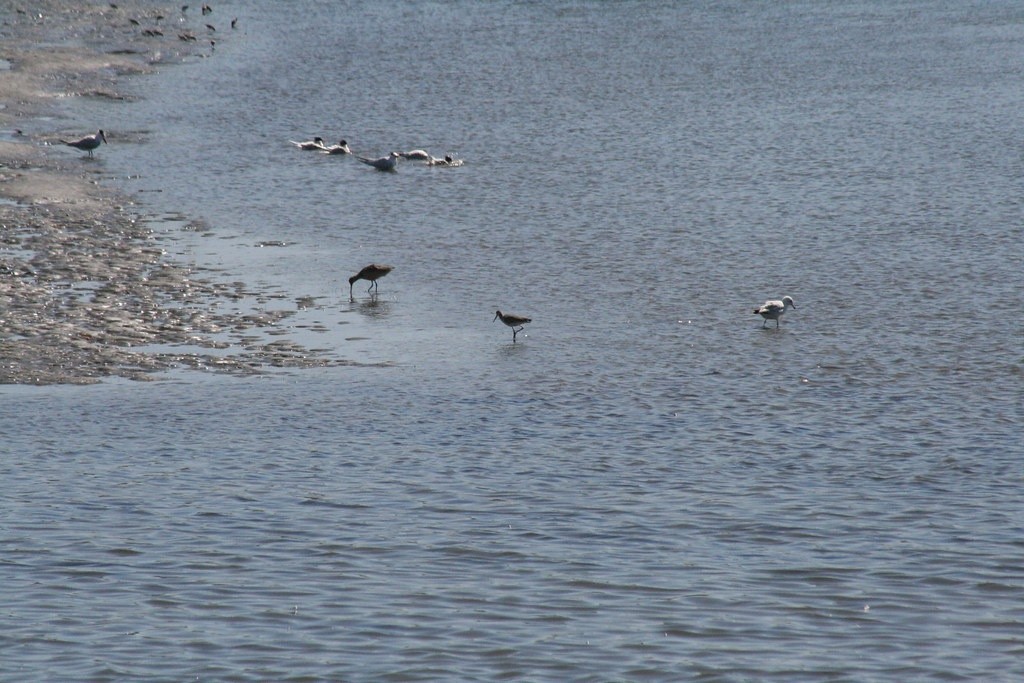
[182,5,190,14]
[426,156,452,166]
[152,29,163,37]
[210,40,216,48]
[108,2,118,11]
[753,295,796,326]
[349,264,396,299]
[205,24,216,31]
[288,137,326,150]
[231,17,238,29]
[60,129,108,157]
[129,18,140,28]
[399,150,429,160]
[156,15,165,24]
[177,33,191,42]
[141,28,155,38]
[493,310,532,339]
[16,8,26,16]
[183,33,196,40]
[319,140,352,154]
[355,152,399,170]
